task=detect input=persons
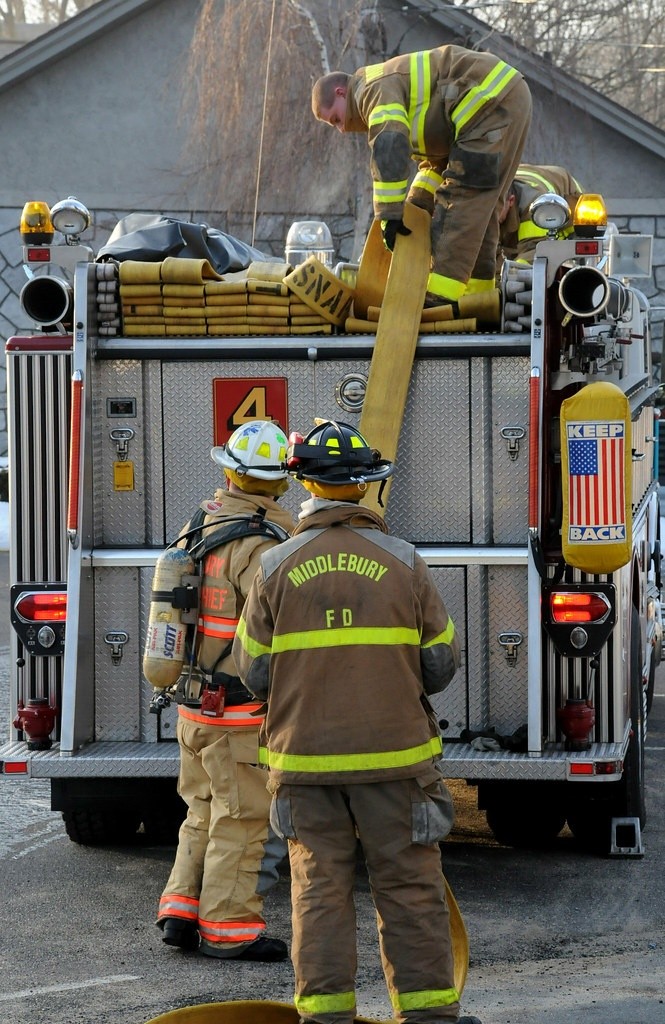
[155,421,294,961]
[234,419,480,1024]
[497,164,583,280]
[310,44,532,306]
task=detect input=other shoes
[233,938,289,964]
[457,1013,485,1024]
[162,918,201,951]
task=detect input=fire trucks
[0,189,664,849]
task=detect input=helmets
[209,421,293,482]
[289,419,396,484]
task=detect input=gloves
[379,218,412,254]
